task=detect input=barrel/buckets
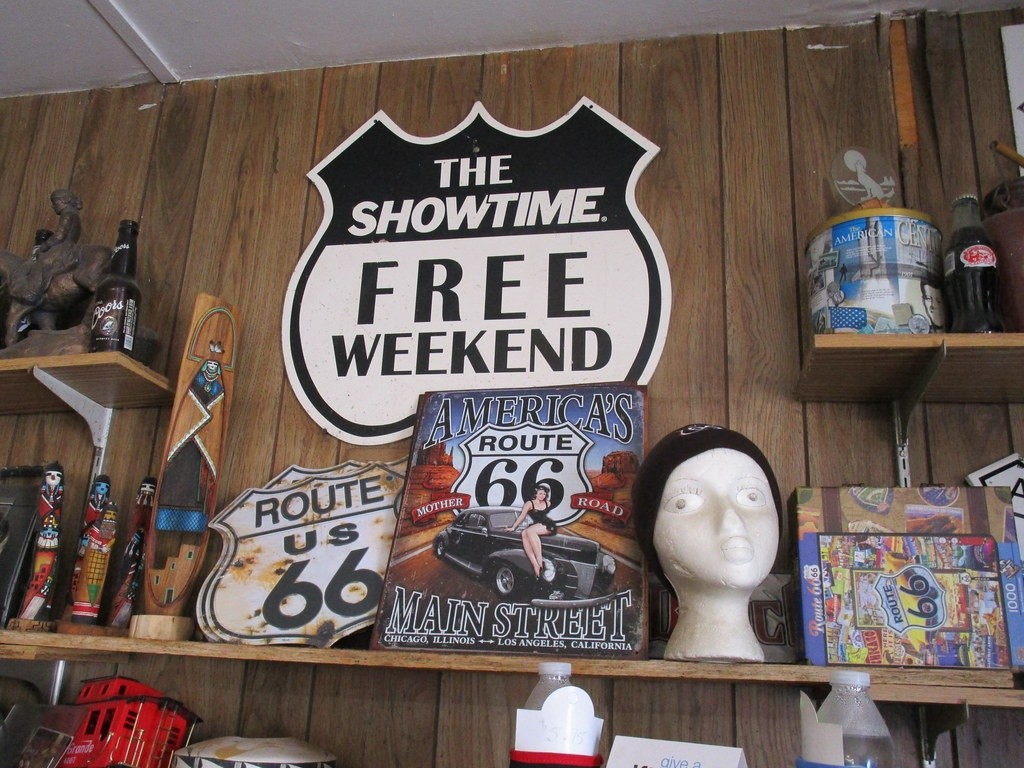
[801,209,943,333]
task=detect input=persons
[14,460,157,627]
[34,189,83,294]
[633,424,783,664]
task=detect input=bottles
[941,194,1006,333]
[89,218,143,351]
[14,230,53,336]
[817,672,894,768]
[524,661,574,710]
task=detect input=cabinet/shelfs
[0,334,1024,715]
